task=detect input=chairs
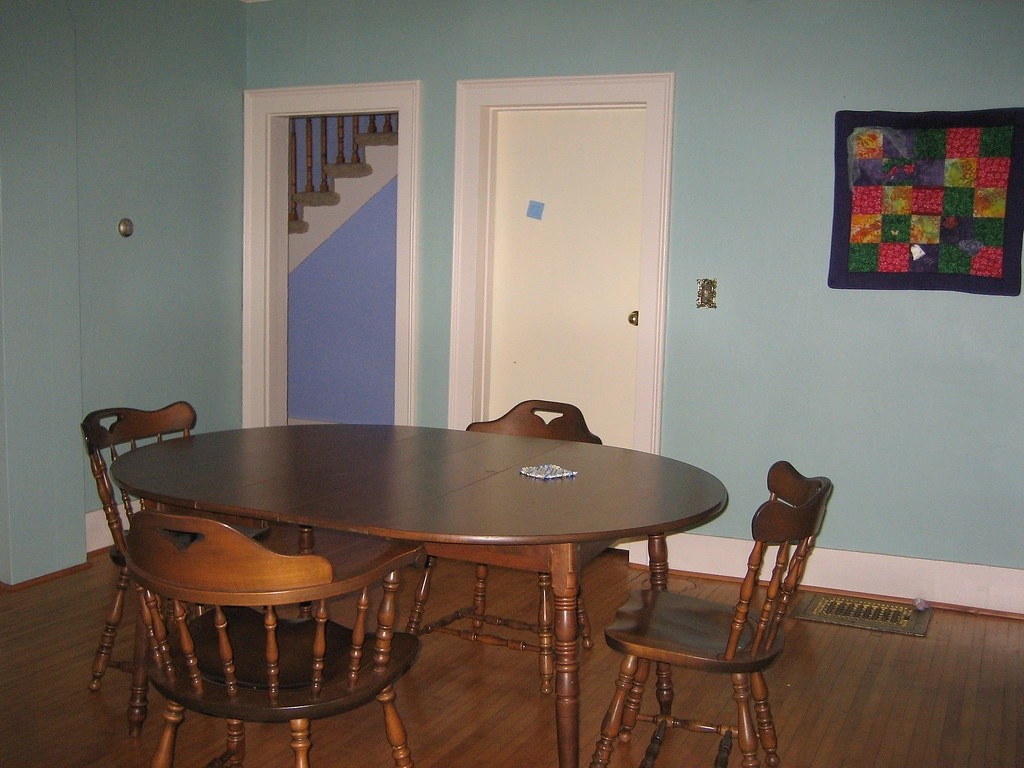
[588,460,834,768]
[405,399,603,694]
[125,508,430,767]
[80,401,198,739]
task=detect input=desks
[108,425,729,767]
